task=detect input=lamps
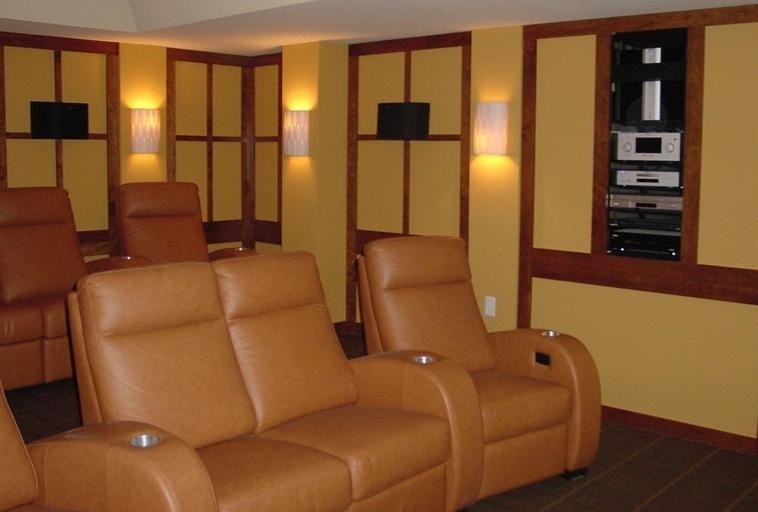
[473,100,508,156]
[282,110,310,157]
[130,108,160,155]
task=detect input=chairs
[356,233,600,498]
[0,382,216,511]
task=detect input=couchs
[61,250,481,511]
